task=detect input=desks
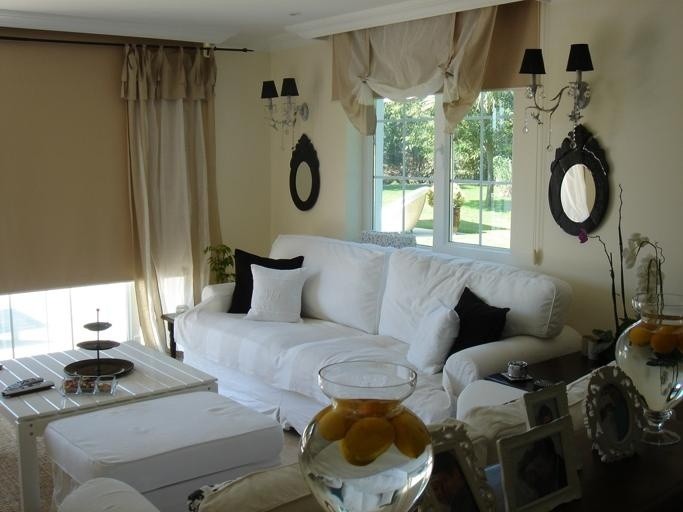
[547,403,683,512]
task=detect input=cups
[62,375,116,396]
[175,304,190,314]
[507,360,528,378]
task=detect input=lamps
[518,44,594,151]
[260,78,308,151]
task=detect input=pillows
[244,263,309,323]
[406,298,459,375]
[227,248,304,312]
[446,288,510,358]
[361,231,416,248]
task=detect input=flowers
[623,233,665,325]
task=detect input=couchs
[54,377,535,512]
[174,234,573,440]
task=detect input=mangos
[630,325,683,355]
[319,399,431,466]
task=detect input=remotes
[4,378,44,390]
[2,381,54,397]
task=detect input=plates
[501,372,533,382]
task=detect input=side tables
[486,350,614,391]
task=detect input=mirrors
[547,124,609,236]
[290,133,320,211]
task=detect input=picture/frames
[524,382,569,428]
[583,366,644,462]
[498,415,583,512]
[422,425,496,512]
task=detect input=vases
[615,294,683,446]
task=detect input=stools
[45,392,284,511]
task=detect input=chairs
[380,188,427,232]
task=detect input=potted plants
[428,183,465,232]
